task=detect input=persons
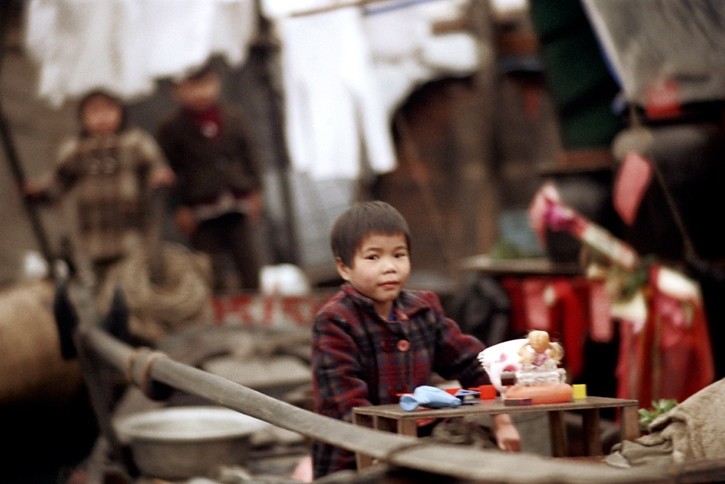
[153,64,266,298]
[312,202,521,484]
[26,88,173,337]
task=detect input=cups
[480,385,496,400]
[572,384,586,399]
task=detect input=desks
[350,395,642,472]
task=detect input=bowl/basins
[121,406,265,478]
